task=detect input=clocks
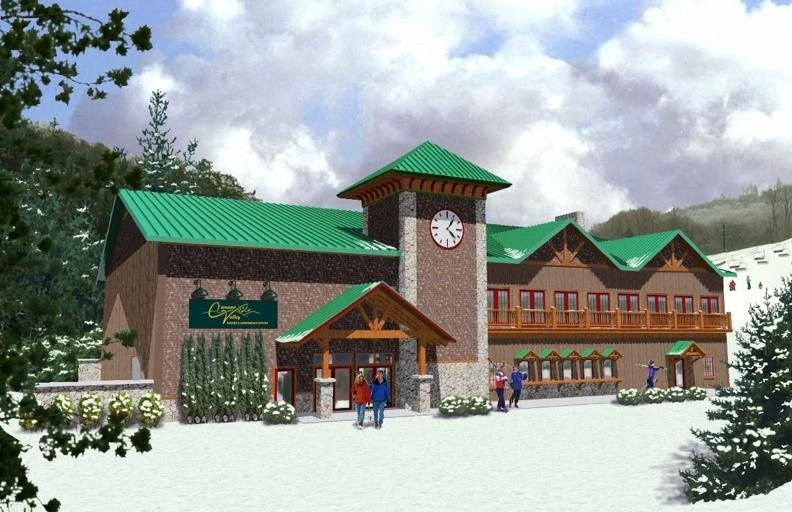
[429,208,464,248]
